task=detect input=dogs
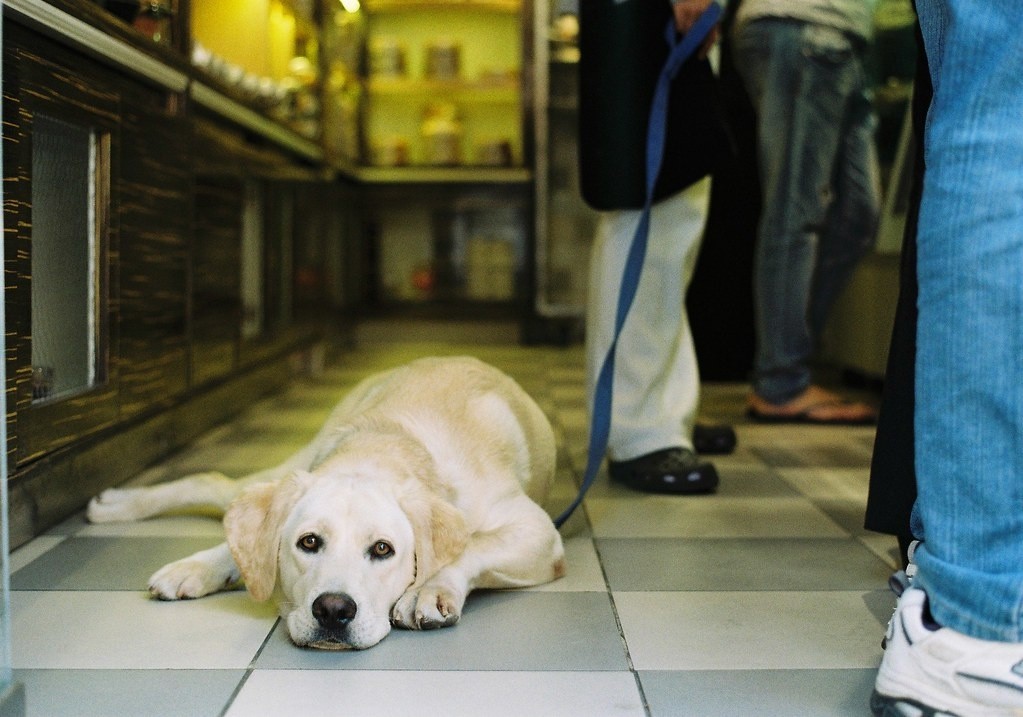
[84,355,567,651]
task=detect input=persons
[580,0,1023,717]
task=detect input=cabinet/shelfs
[0,0,593,551]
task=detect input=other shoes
[869,570,1023,717]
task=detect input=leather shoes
[609,447,718,495]
[693,422,736,455]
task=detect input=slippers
[747,384,876,424]
